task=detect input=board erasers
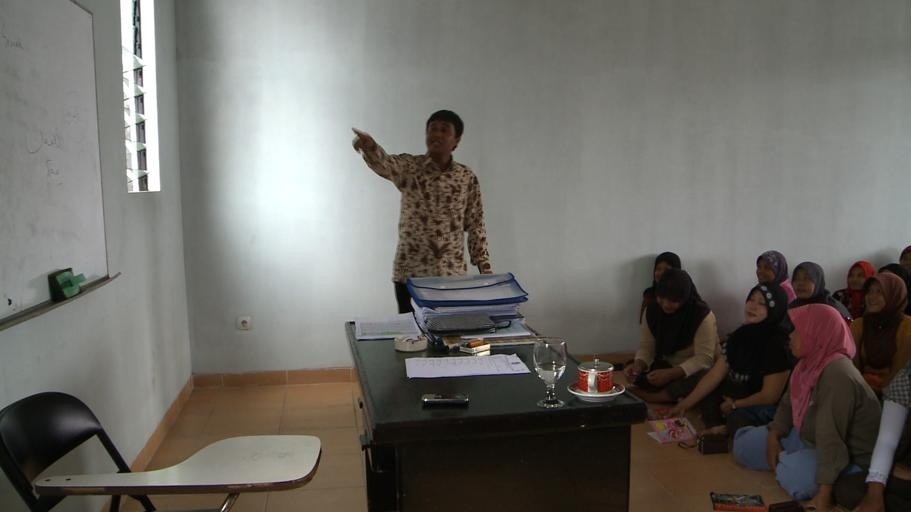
[48,268,86,302]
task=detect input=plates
[566,382,627,405]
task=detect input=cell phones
[421,394,469,406]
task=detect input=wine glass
[532,336,570,409]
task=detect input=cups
[578,357,616,394]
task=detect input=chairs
[0,392,222,512]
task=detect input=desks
[346,321,649,512]
[35,435,322,512]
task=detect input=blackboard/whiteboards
[0,0,122,331]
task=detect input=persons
[347,110,498,314]
[625,248,911,512]
